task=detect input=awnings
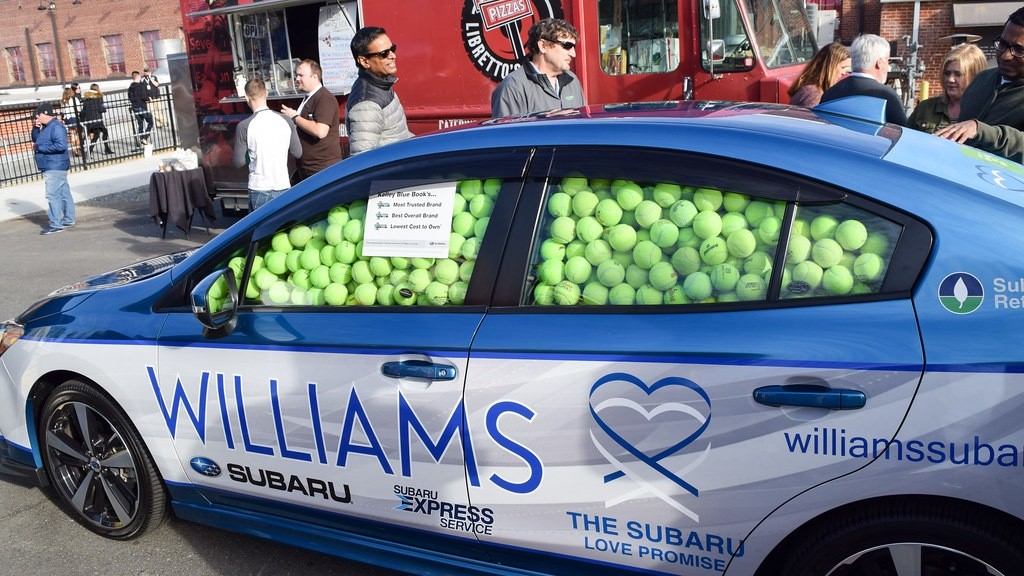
[953,2,1024,27]
[185,0,356,46]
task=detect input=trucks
[178,0,849,218]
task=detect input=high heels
[105,148,115,154]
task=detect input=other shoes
[140,139,148,145]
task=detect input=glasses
[365,45,396,58]
[992,34,1024,58]
[543,37,576,50]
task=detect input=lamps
[37,0,51,10]
[73,0,81,5]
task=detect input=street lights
[18,0,82,97]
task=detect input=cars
[0,101,1024,576]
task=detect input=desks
[148,166,216,241]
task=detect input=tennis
[208,276,229,314]
[228,174,897,307]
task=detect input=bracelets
[292,115,298,121]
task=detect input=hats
[37,103,53,115]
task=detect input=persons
[787,42,851,107]
[907,44,987,134]
[31,103,75,235]
[491,18,586,118]
[128,70,154,145]
[140,67,167,129]
[60,82,83,156]
[281,59,343,180]
[819,33,906,128]
[233,78,302,214]
[83,84,115,159]
[345,26,415,155]
[931,7,1024,165]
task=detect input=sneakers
[63,222,75,226]
[42,227,63,234]
